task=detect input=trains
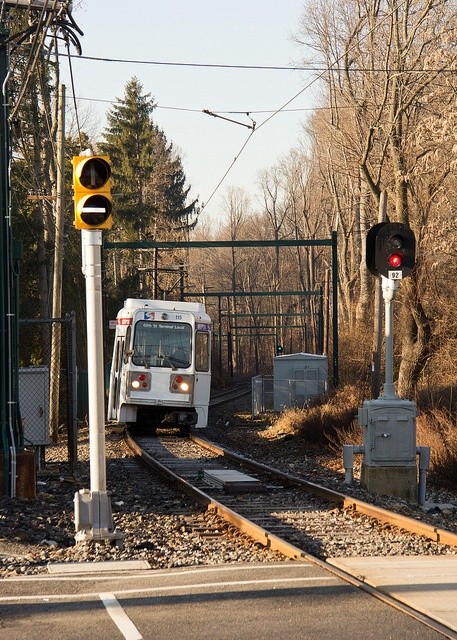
[106,297,212,434]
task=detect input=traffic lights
[277,346,284,353]
[71,155,113,229]
[375,222,415,281]
